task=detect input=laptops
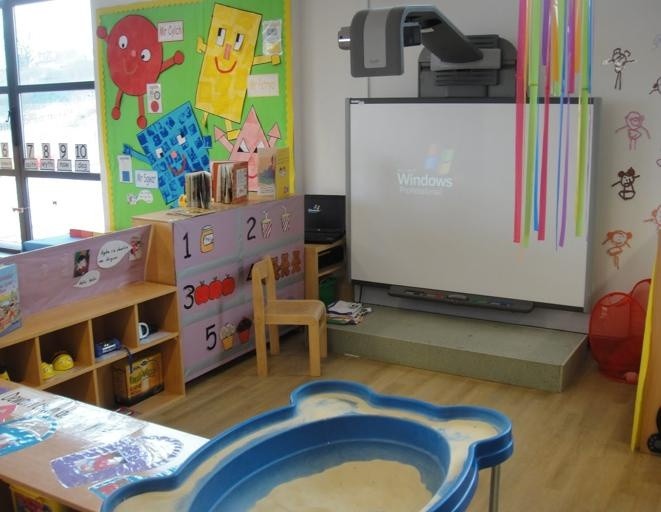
[304,195,345,244]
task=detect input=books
[326,300,372,325]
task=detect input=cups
[139,320,149,340]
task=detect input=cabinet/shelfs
[0,280,228,512]
[304,238,346,307]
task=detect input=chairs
[252,255,329,379]
